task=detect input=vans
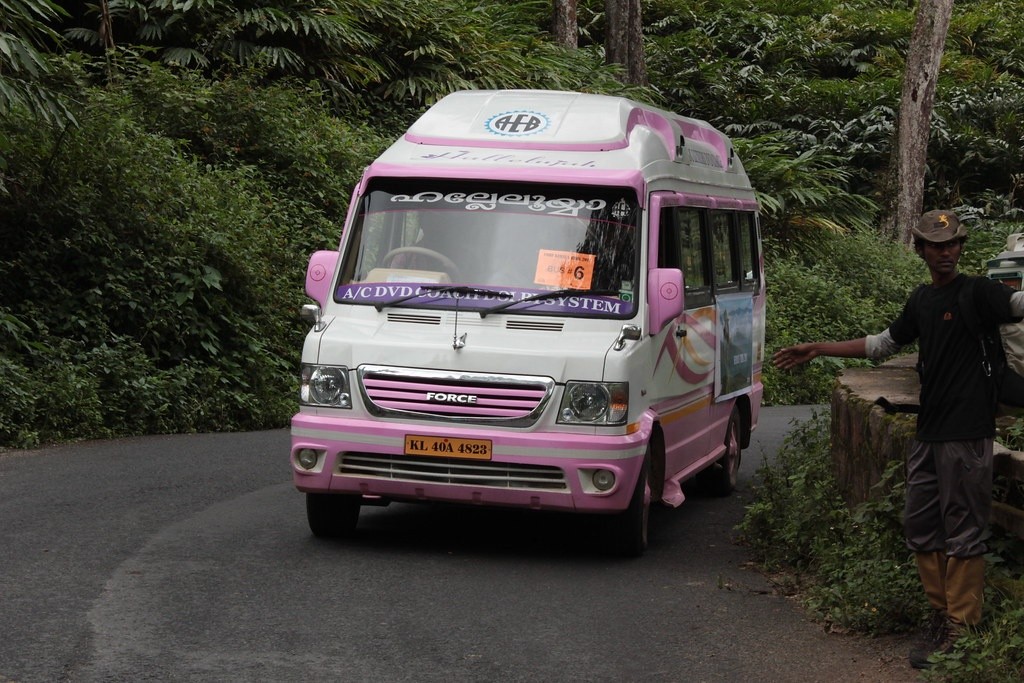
[289,88,768,558]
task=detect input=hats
[911,209,967,243]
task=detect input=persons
[389,211,466,274]
[486,214,612,293]
[771,208,1024,670]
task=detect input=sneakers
[909,624,966,668]
[907,622,939,655]
